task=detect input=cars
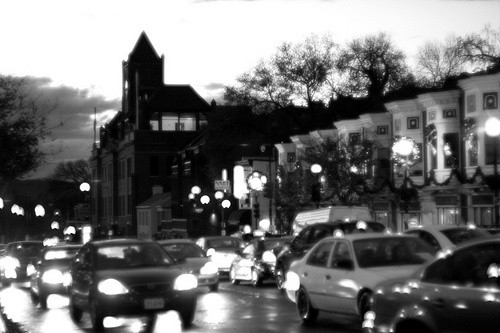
[399,224,493,261]
[64,238,202,329]
[4,239,44,290]
[273,218,387,305]
[156,239,218,292]
[196,228,298,291]
[367,238,500,331]
[284,233,458,326]
[28,244,89,311]
[0,244,16,284]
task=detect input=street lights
[181,107,500,237]
